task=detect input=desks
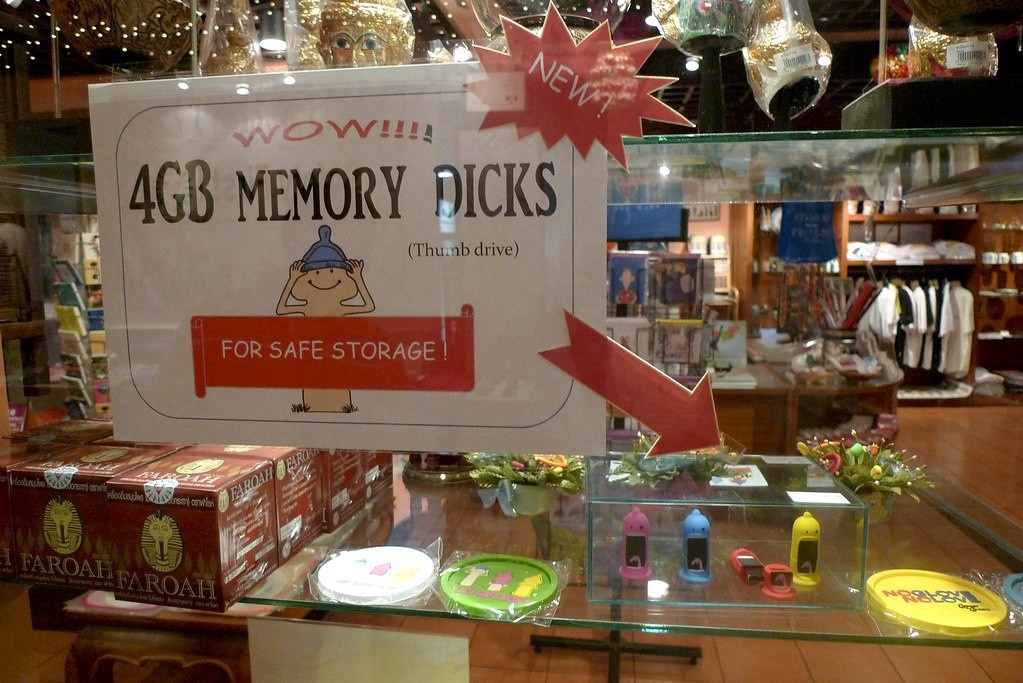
[26,455,1023,683]
[605,353,906,456]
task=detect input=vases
[512,483,551,515]
[856,493,893,525]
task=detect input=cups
[982,251,1023,265]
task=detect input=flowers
[464,450,585,517]
[797,430,933,504]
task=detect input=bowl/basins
[998,289,1018,296]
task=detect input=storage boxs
[2,417,396,611]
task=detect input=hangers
[867,275,961,289]
